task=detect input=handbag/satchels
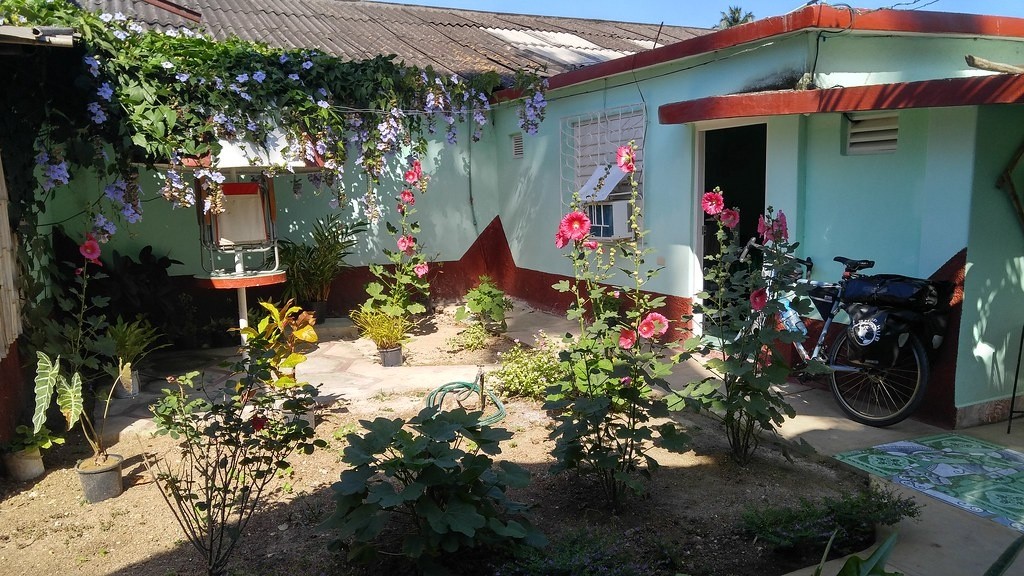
[844,273,947,312]
[846,301,904,368]
[897,316,952,375]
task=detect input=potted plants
[98,315,176,397]
[0,423,65,487]
[263,214,370,324]
[33,351,136,503]
[351,305,414,367]
[226,292,317,432]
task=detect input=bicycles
[738,236,940,429]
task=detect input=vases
[45,377,98,431]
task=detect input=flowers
[29,232,107,383]
